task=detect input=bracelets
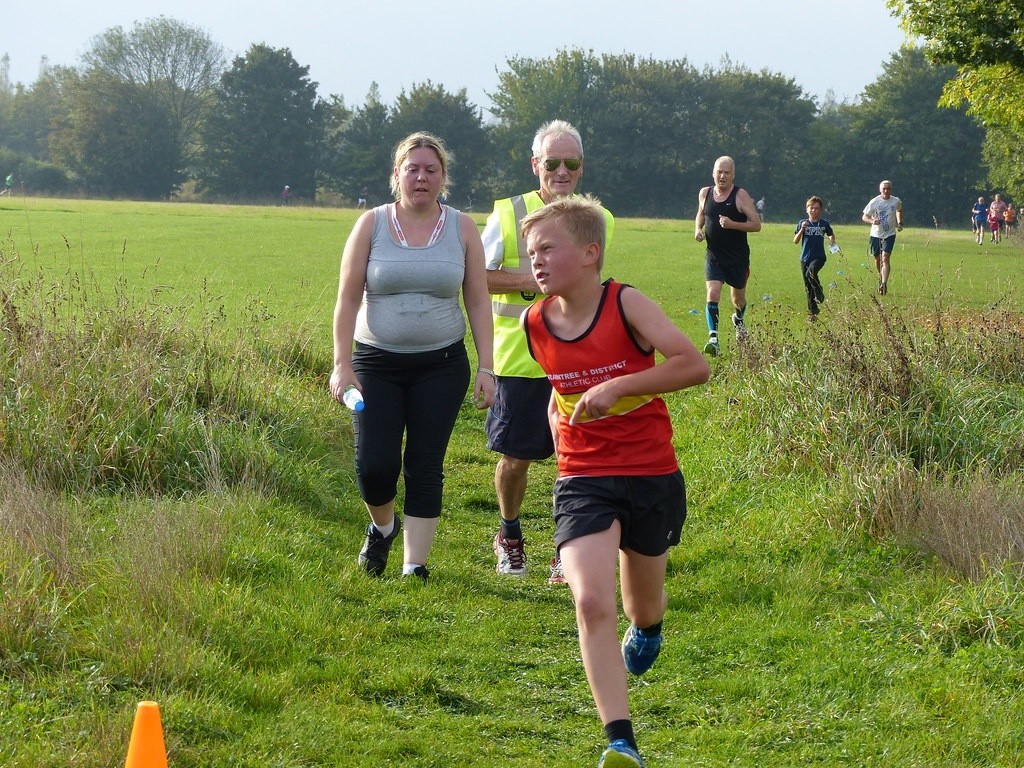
[477,367,497,386]
[897,221,903,226]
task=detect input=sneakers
[359,514,401,578]
[547,563,567,584]
[598,739,642,768]
[402,566,430,586]
[493,531,527,576]
[621,622,662,676]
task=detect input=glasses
[536,159,580,170]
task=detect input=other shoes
[877,284,886,295]
[704,342,720,357]
[815,287,824,303]
[732,313,748,341]
[808,316,817,324]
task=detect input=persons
[756,196,765,223]
[329,133,497,581]
[519,201,710,768]
[825,201,833,224]
[468,187,477,213]
[793,196,835,323]
[282,185,290,207]
[357,186,367,209]
[694,156,761,358]
[971,194,1024,245]
[441,190,449,205]
[481,120,614,588]
[862,180,903,295]
[0,172,14,198]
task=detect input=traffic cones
[124,701,171,768]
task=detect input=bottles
[343,384,365,411]
